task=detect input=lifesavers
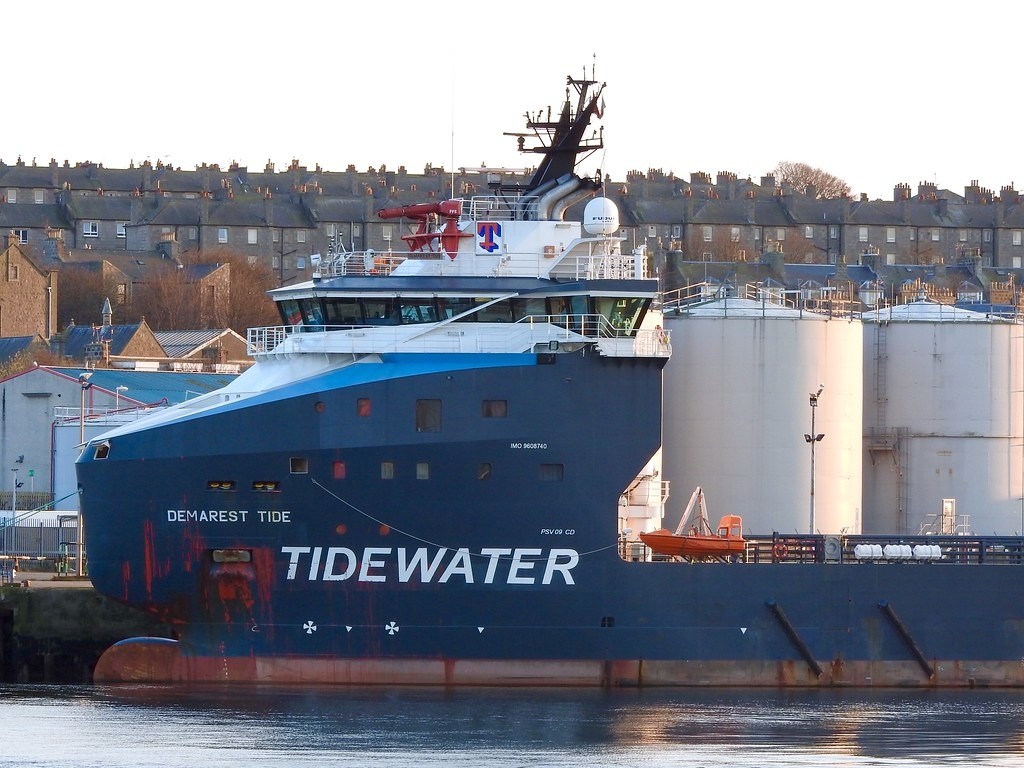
[772,543,789,559]
[655,324,668,345]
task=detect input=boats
[638,487,746,555]
[75,52,1024,684]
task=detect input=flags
[601,97,606,116]
[592,90,600,119]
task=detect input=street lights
[116,387,129,415]
[11,469,24,572]
[76,372,93,576]
[804,389,825,550]
